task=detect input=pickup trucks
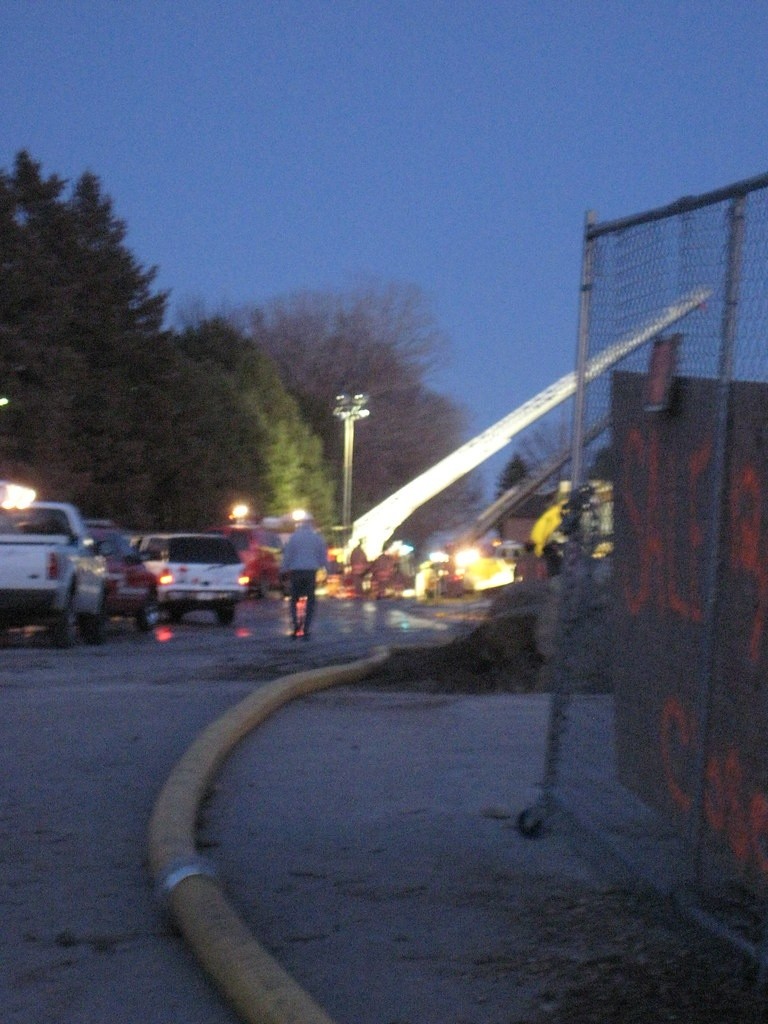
[0,502,107,647]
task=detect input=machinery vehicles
[418,410,611,600]
[312,276,715,601]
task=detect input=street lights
[332,392,371,550]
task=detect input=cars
[80,520,165,633]
[233,546,284,600]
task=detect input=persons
[282,513,329,639]
[512,541,548,582]
[537,541,564,577]
[328,538,408,602]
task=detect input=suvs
[125,531,250,625]
